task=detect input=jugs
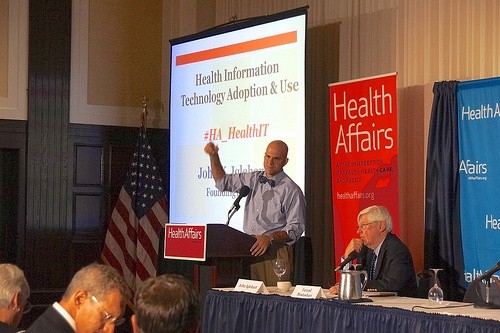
[338,269,368,300]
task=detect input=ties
[369,251,377,280]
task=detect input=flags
[101,123,169,312]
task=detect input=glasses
[87,290,125,326]
[357,219,378,227]
[21,293,33,314]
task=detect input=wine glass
[273,259,286,281]
[350,263,362,288]
[428,268,444,304]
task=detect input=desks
[202,286,500,333]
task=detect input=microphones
[335,250,358,272]
[476,261,500,281]
[229,185,250,214]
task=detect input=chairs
[416,270,441,299]
[463,275,500,306]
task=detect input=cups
[277,282,291,292]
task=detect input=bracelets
[266,234,273,244]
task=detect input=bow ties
[259,176,275,188]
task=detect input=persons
[204,140,305,286]
[131,273,199,333]
[0,263,30,333]
[330,205,418,298]
[23,263,131,333]
